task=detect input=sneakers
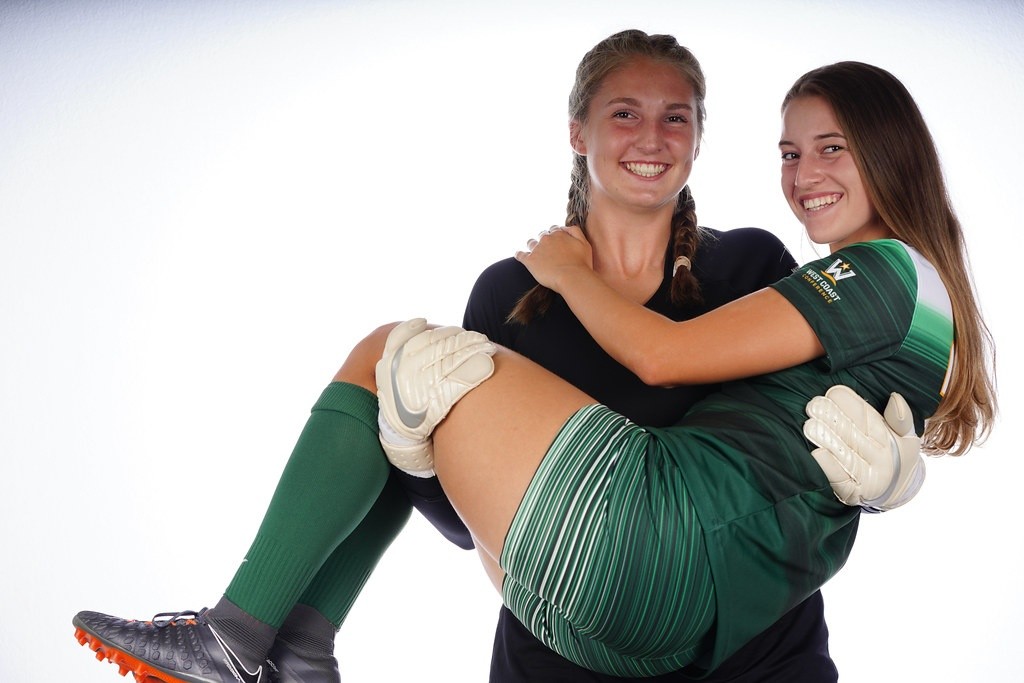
[72,610,279,683]
[268,637,340,683]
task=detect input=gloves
[376,319,498,479]
[803,384,926,513]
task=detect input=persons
[375,30,925,683]
[73,62,998,683]
